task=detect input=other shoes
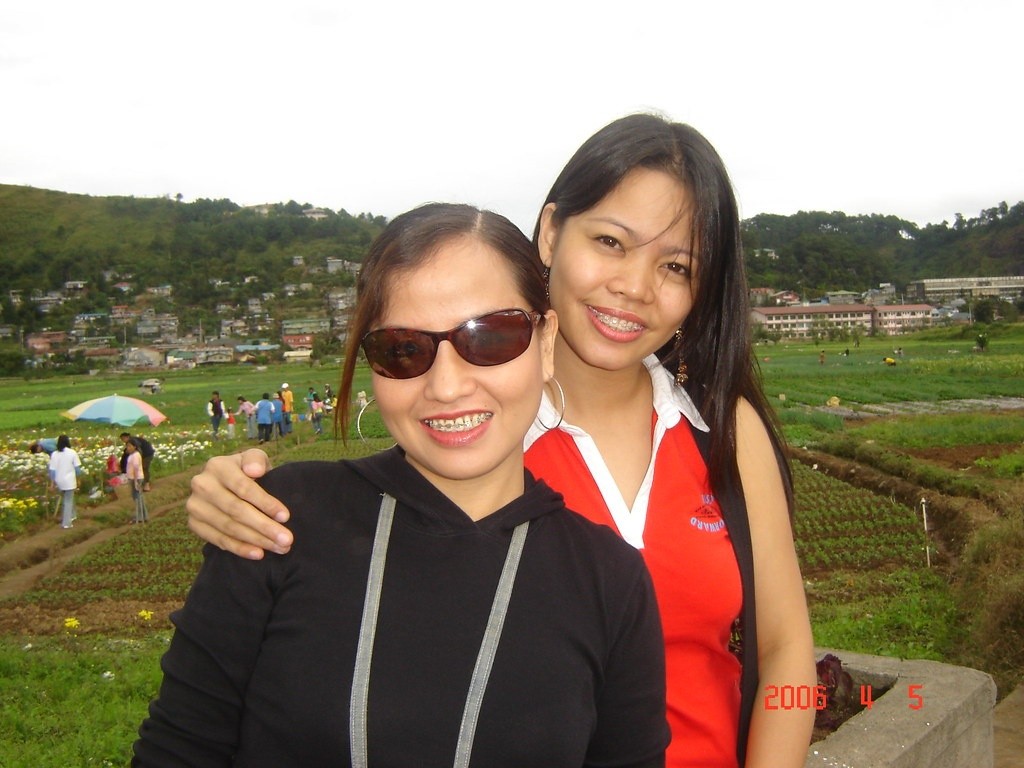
[63,524,73,528]
[127,520,150,523]
[314,428,321,434]
[70,516,78,521]
[259,439,263,444]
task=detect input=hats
[281,382,289,388]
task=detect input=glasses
[361,307,544,380]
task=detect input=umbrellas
[59,393,167,463]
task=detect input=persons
[311,396,325,435]
[844,348,849,356]
[207,391,228,442]
[30,439,81,489]
[306,387,317,419]
[883,357,896,368]
[898,347,904,357]
[254,393,275,444]
[272,393,285,441]
[282,382,293,434]
[323,383,337,415]
[819,350,825,366]
[235,396,256,439]
[50,436,81,528]
[120,432,154,492]
[131,203,672,768]
[125,440,148,523]
[277,391,286,436]
[185,115,818,768]
[227,407,236,439]
